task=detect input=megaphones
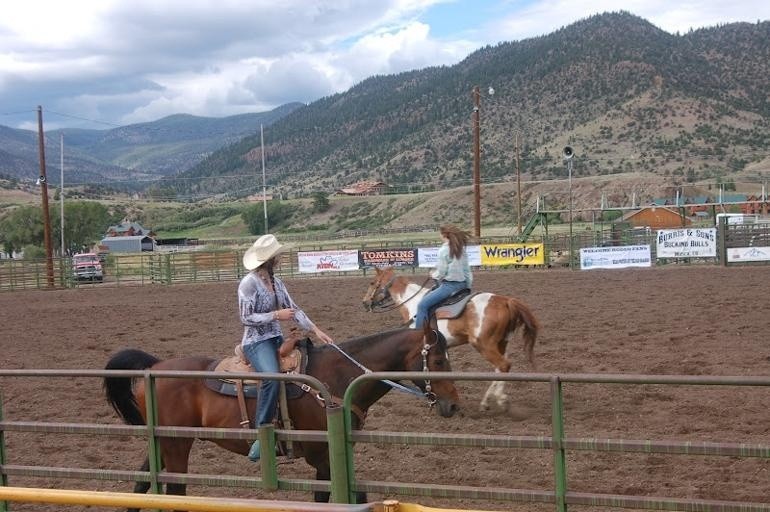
[562,145,574,159]
[39,176,46,181]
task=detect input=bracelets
[275,309,279,320]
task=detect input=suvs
[72,253,104,281]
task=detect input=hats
[242,234,295,271]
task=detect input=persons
[237,234,333,462]
[416,224,478,332]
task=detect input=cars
[98,253,109,262]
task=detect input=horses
[362,265,539,413]
[100,313,461,512]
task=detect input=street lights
[561,146,577,271]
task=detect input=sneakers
[248,441,260,461]
